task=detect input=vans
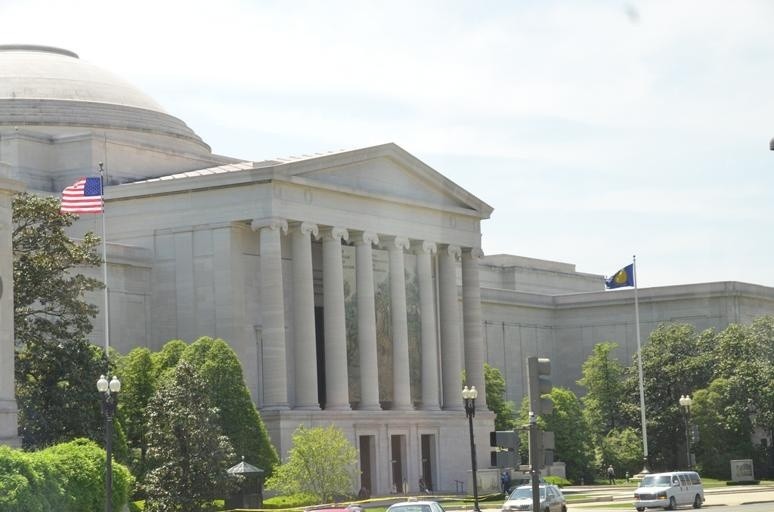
[632,470,706,512]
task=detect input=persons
[418,478,433,495]
[499,465,514,496]
[358,483,371,499]
[607,464,617,485]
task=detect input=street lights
[96,373,122,512]
[679,392,693,472]
[460,384,484,512]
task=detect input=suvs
[500,482,567,512]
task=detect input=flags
[59,176,105,215]
[605,263,634,288]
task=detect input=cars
[383,497,446,512]
[302,503,364,512]
